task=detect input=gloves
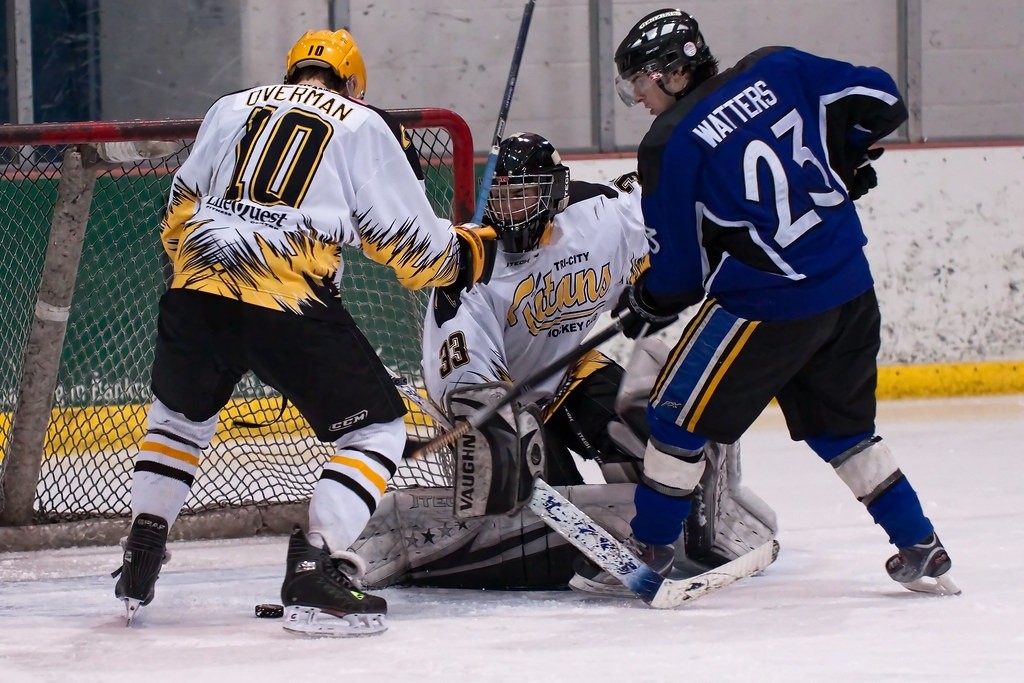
[839,158,877,200]
[611,286,679,340]
[454,222,497,293]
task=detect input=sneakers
[111,513,168,627]
[568,533,675,599]
[885,530,963,596]
[281,530,389,638]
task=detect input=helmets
[286,29,366,100]
[614,8,705,80]
[482,132,570,261]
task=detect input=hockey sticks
[400,317,623,459]
[377,348,781,612]
[472,1,536,226]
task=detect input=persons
[570,7,952,586]
[111,25,498,619]
[338,128,778,598]
